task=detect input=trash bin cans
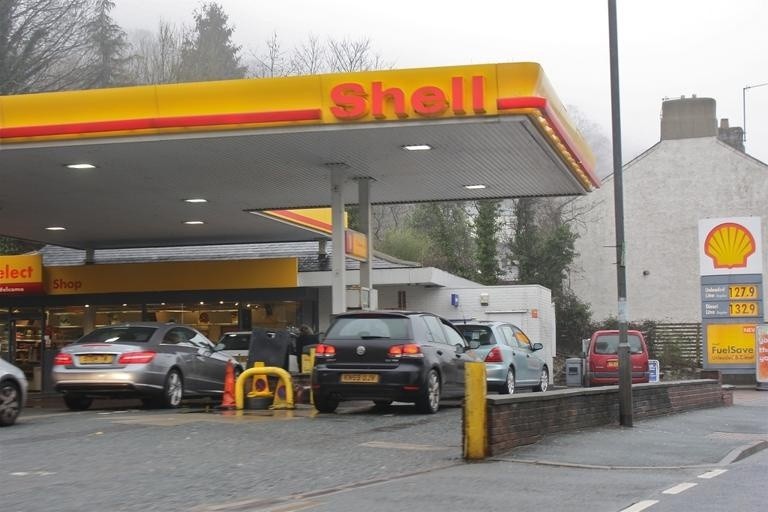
[566,358,583,388]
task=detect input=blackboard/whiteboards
[646,360,658,382]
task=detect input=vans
[585,330,649,387]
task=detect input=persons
[296,324,320,372]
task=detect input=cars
[313,311,549,412]
[0,322,276,427]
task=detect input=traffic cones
[214,359,235,411]
